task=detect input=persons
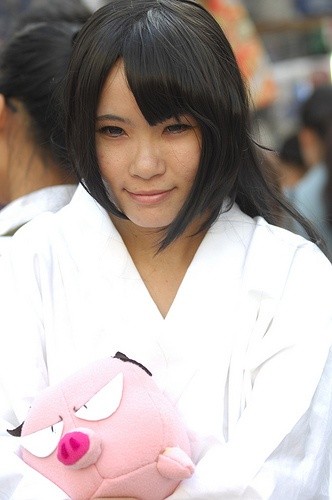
[0,0,90,245]
[243,40,330,260]
[1,1,330,500]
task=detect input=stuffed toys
[6,351,205,499]
